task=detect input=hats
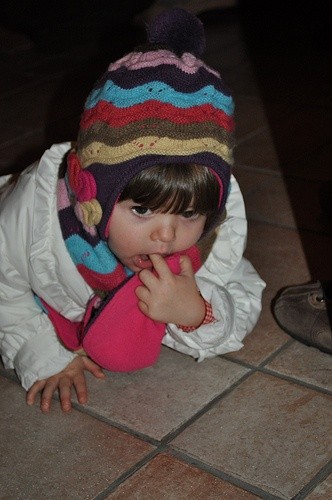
[65,7,237,248]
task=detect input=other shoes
[272,282,332,354]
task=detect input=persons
[237,1,332,355]
[0,48,266,412]
